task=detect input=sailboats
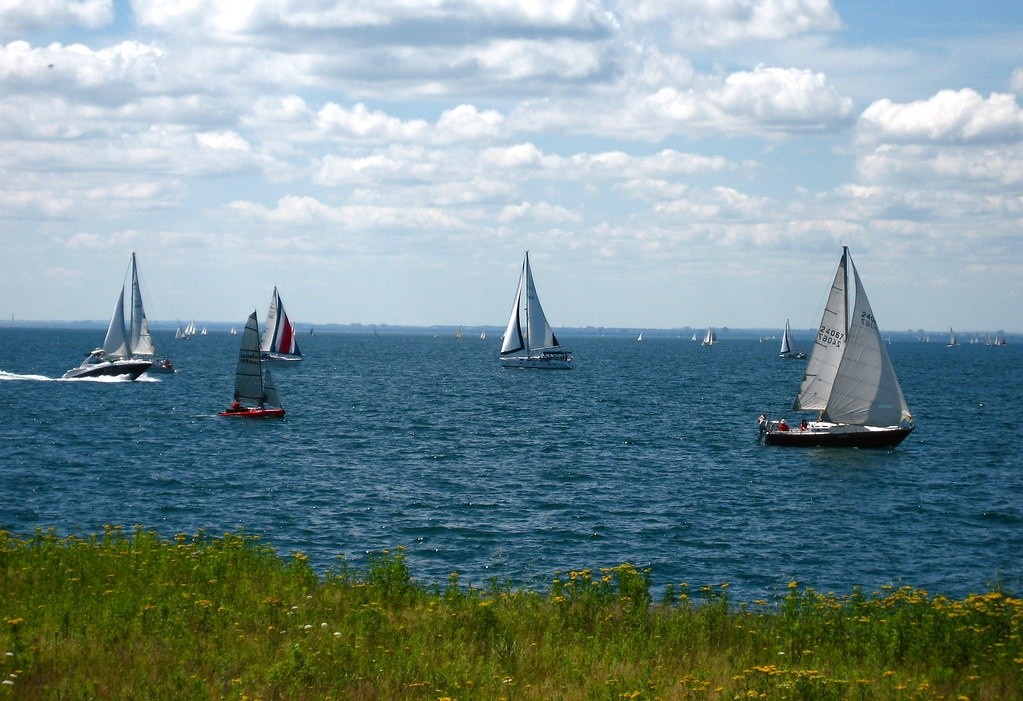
[173,318,207,340]
[256,286,303,362]
[61,251,176,380]
[778,317,808,360]
[945,327,1009,349]
[757,243,916,449]
[701,326,720,347]
[217,309,286,417]
[498,250,575,369]
